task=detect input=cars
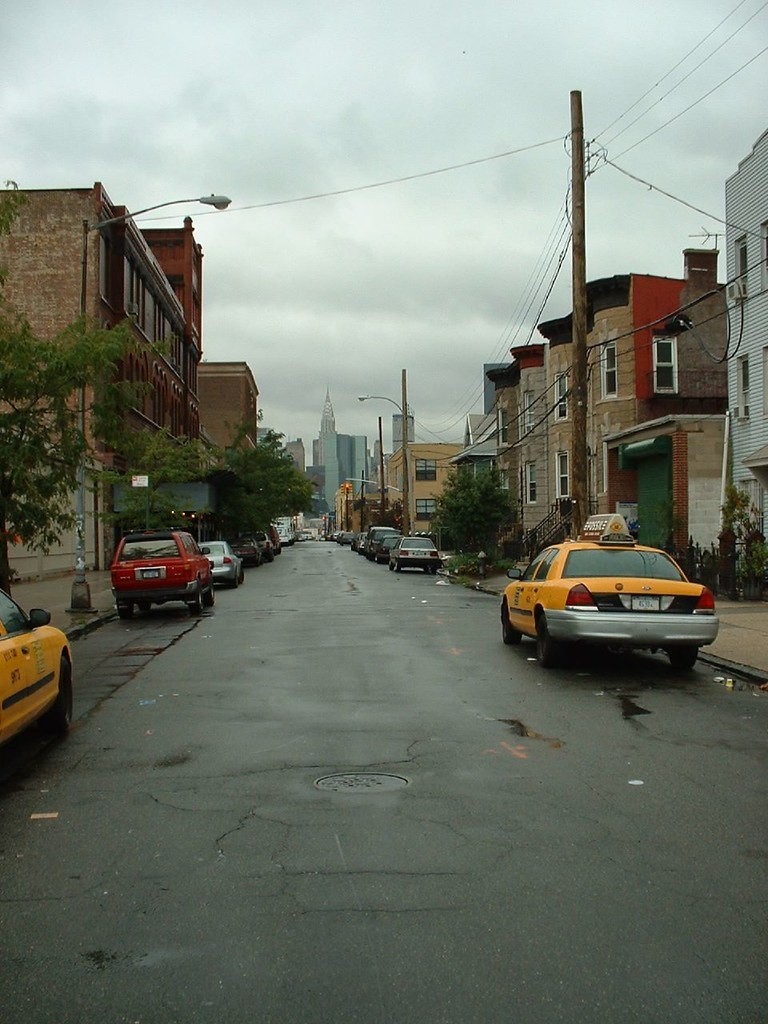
[197,541,245,589]
[319,527,405,565]
[0,587,75,747]
[388,537,440,575]
[500,540,719,672]
[230,517,314,566]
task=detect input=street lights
[65,193,232,613]
[358,396,411,537]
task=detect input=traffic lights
[341,482,346,493]
[347,483,352,494]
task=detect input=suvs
[110,527,215,622]
